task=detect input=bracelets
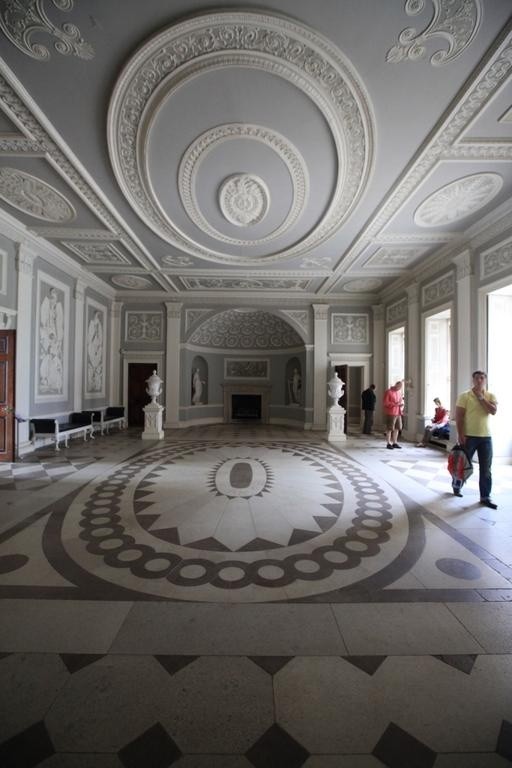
[479,397,484,401]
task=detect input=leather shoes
[387,442,402,449]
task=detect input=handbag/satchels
[448,444,472,481]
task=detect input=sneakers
[479,498,497,509]
[452,487,463,497]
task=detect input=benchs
[426,422,450,451]
[30,406,126,451]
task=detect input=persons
[293,368,300,400]
[362,384,376,434]
[416,397,448,447]
[452,371,498,509]
[383,381,404,449]
[192,367,206,402]
